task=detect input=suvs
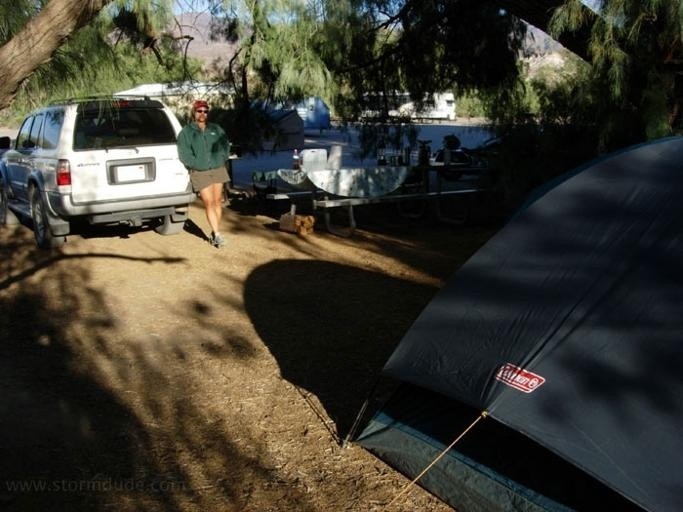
[0,93,191,249]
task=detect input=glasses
[195,110,209,114]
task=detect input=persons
[175,100,231,250]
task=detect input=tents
[331,132,682,510]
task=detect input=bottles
[291,149,300,171]
[375,144,410,166]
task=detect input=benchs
[263,183,480,239]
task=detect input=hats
[194,100,210,109]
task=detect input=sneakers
[209,231,228,249]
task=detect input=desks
[252,163,468,240]
